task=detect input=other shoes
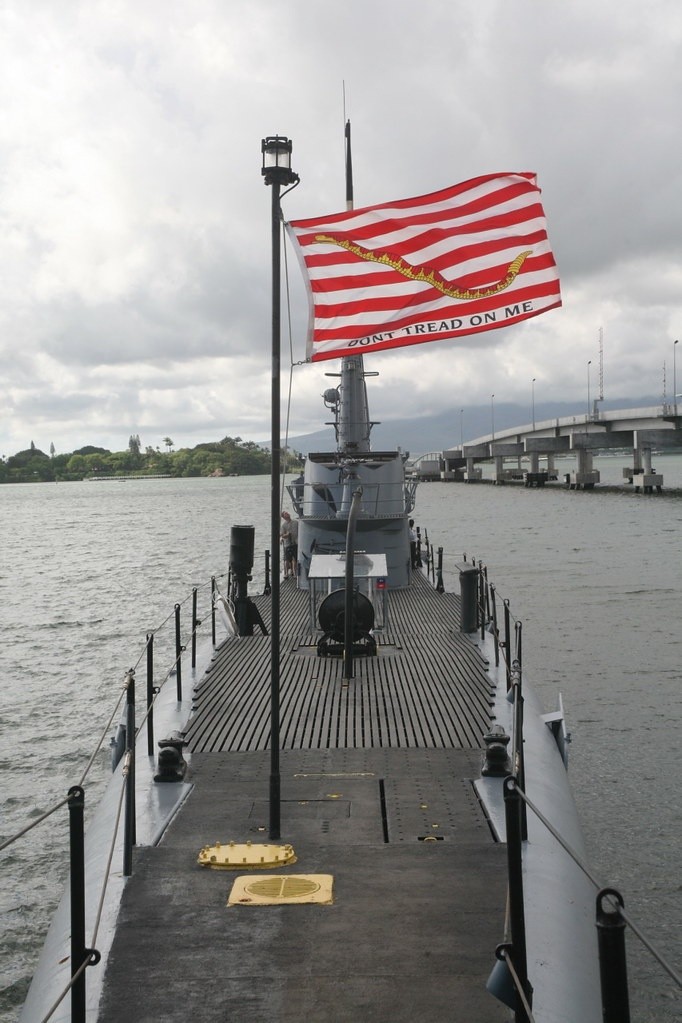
[412,565,418,568]
[284,575,289,579]
[294,575,297,579]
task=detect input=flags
[284,172,562,364]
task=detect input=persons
[280,511,298,579]
[408,519,420,569]
[291,471,304,499]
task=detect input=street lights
[587,360,592,435]
[491,394,494,444]
[258,134,301,839]
[532,378,536,438]
[674,339,679,417]
[460,409,464,449]
